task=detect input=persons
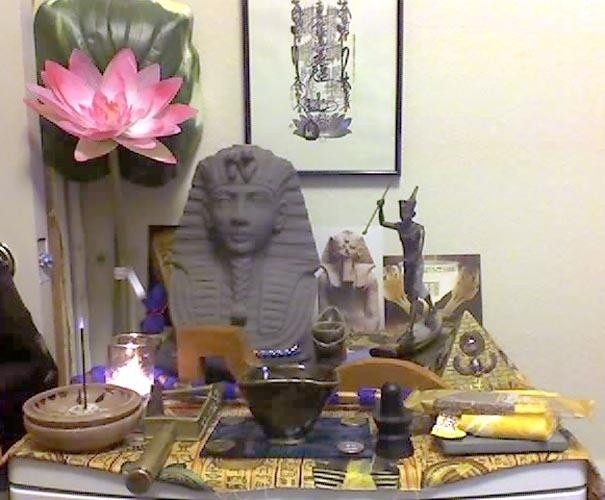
[375,197,434,345]
[317,228,381,329]
[166,142,322,372]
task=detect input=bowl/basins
[25,383,141,453]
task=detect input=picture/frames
[239,0,405,178]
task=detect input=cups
[103,332,157,410]
[238,362,339,446]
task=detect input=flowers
[23,42,203,336]
